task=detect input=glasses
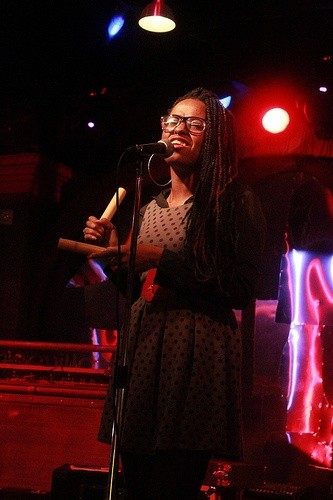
[160,114,209,136]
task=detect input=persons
[83,88,256,500]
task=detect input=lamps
[136,0,178,34]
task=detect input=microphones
[135,140,174,158]
